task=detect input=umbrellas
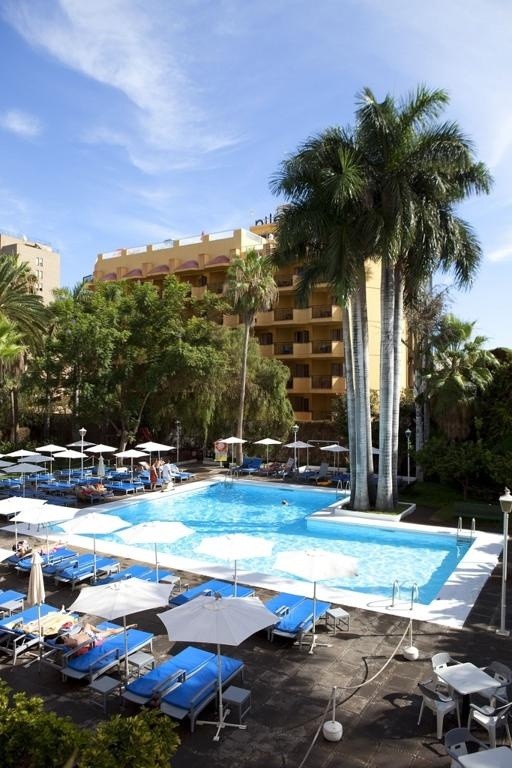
[0,439,176,498]
[26,551,46,660]
[66,575,176,678]
[56,512,132,578]
[271,546,359,644]
[113,520,196,585]
[194,533,274,598]
[154,593,282,723]
[8,504,80,562]
[218,435,349,475]
[0,495,48,553]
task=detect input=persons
[55,622,138,649]
[282,500,288,507]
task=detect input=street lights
[291,423,299,474]
[78,426,87,479]
[497,486,512,637]
[405,429,411,486]
[174,418,180,466]
